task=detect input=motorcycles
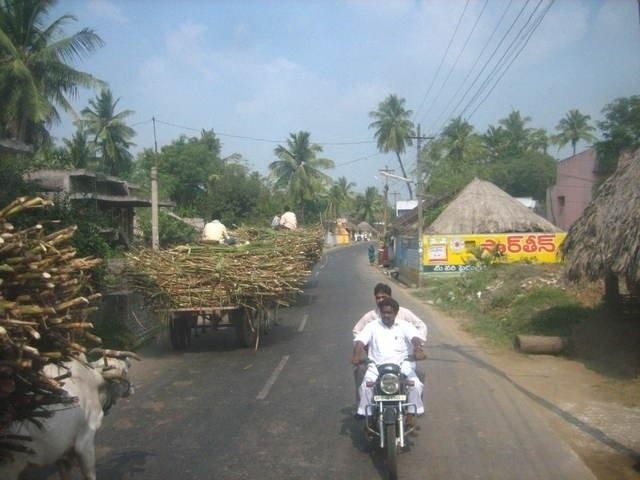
[352,355,417,480]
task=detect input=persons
[271,205,298,230]
[350,231,372,242]
[368,244,375,267]
[351,283,428,420]
[202,211,235,245]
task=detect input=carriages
[154,287,289,351]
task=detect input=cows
[0,348,142,480]
